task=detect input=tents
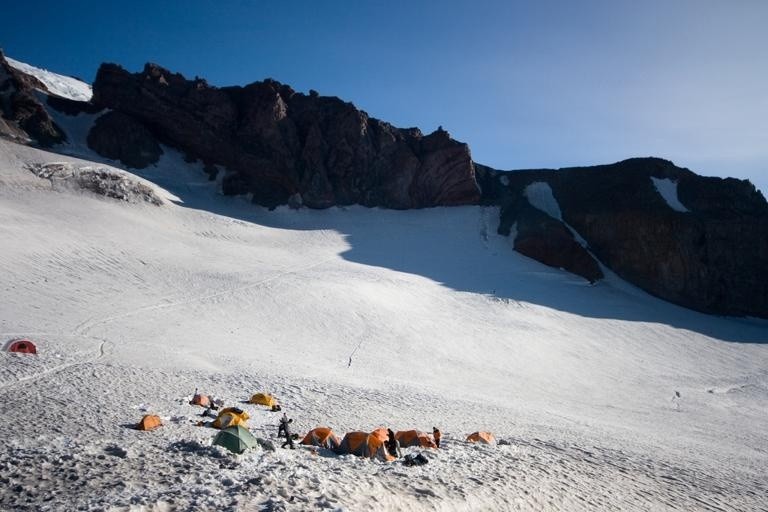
[139,414,162,432]
[466,430,495,445]
[251,391,275,406]
[394,428,438,448]
[298,427,340,449]
[192,393,210,406]
[219,406,250,422]
[213,423,260,454]
[1,337,38,355]
[331,425,403,462]
[212,411,244,429]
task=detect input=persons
[312,436,324,447]
[195,388,199,395]
[283,413,287,423]
[278,417,293,437]
[386,428,398,457]
[281,433,298,449]
[395,439,403,459]
[433,427,441,448]
